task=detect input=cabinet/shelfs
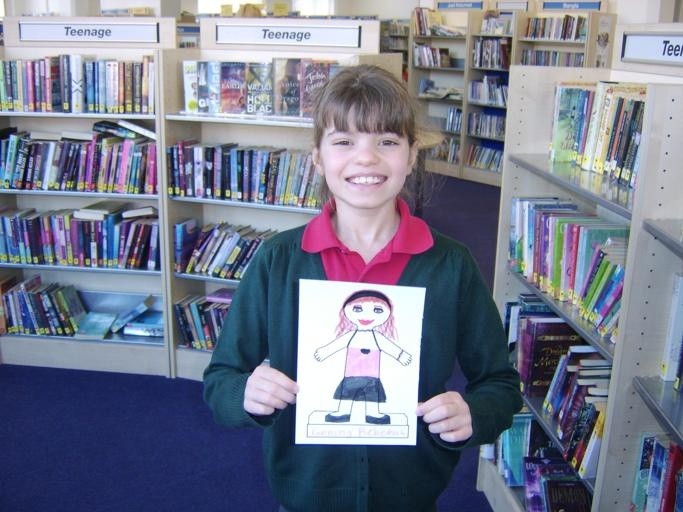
[473,65,682,510]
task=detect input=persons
[200,63,525,512]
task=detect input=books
[1,55,163,355]
[380,6,465,167]
[167,54,355,356]
[628,274,683,512]
[465,14,588,172]
[481,81,649,512]
